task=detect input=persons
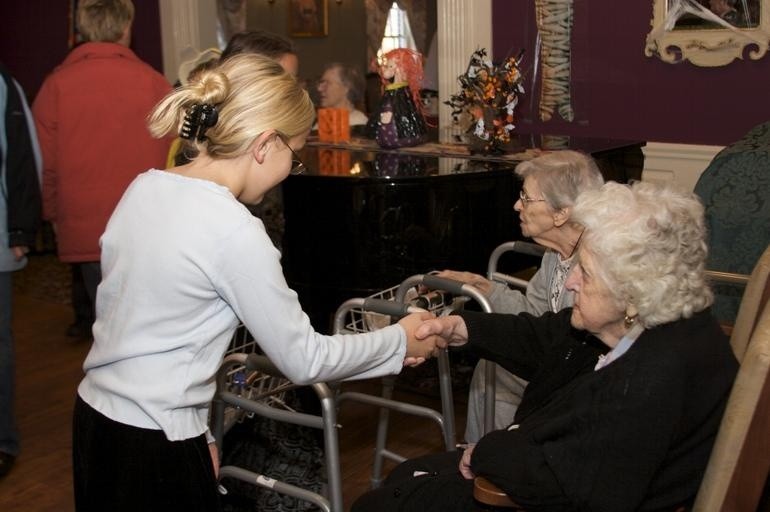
[73,55,447,511]
[350,178,741,512]
[173,29,303,258]
[31,0,178,341]
[0,69,44,483]
[312,58,370,129]
[420,150,605,442]
[372,2,428,147]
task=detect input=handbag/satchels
[223,410,325,511]
[366,82,431,149]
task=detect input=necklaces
[565,227,585,259]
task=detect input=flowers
[445,49,525,138]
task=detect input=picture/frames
[286,0,330,39]
[642,0,770,68]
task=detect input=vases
[466,133,496,157]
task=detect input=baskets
[345,270,465,334]
[208,325,291,424]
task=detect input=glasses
[520,191,545,204]
[278,134,305,175]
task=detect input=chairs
[697,249,769,361]
[471,243,769,512]
